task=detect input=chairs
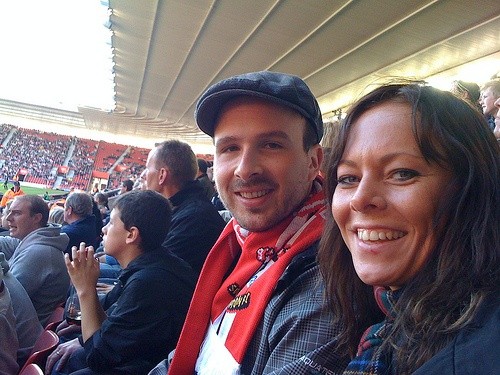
[18,307,65,375]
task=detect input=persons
[320,86,498,374]
[39,135,93,190]
[0,194,71,375]
[0,124,38,189]
[64,189,197,375]
[149,71,385,375]
[45,141,228,375]
[0,181,24,207]
[61,190,99,266]
[452,79,500,138]
[0,162,226,375]
[94,138,214,162]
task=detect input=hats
[194,71,324,144]
[14,181,20,186]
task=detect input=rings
[57,352,59,354]
